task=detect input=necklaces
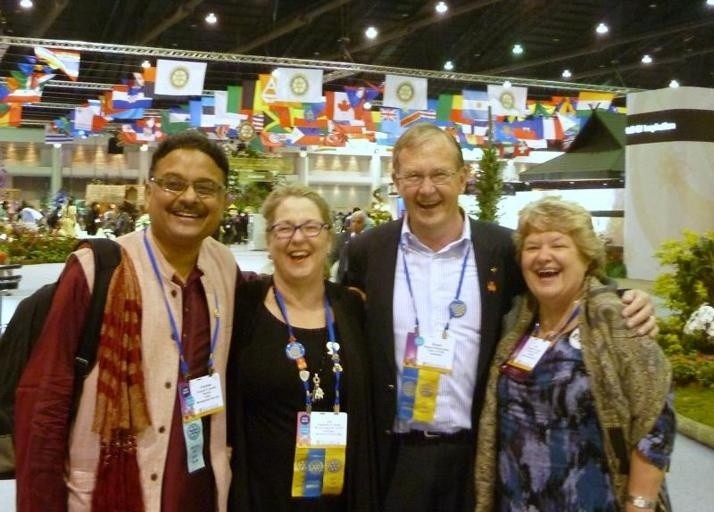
[273,286,329,401]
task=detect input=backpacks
[0,282,58,482]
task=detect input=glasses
[393,164,461,186]
[148,173,228,197]
[264,220,331,240]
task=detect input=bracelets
[626,494,656,510]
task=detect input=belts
[389,428,474,444]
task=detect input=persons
[472,196,680,510]
[337,125,663,510]
[211,210,249,246]
[226,185,378,512]
[328,208,376,263]
[0,188,153,238]
[13,132,368,512]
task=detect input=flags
[1,41,625,157]
